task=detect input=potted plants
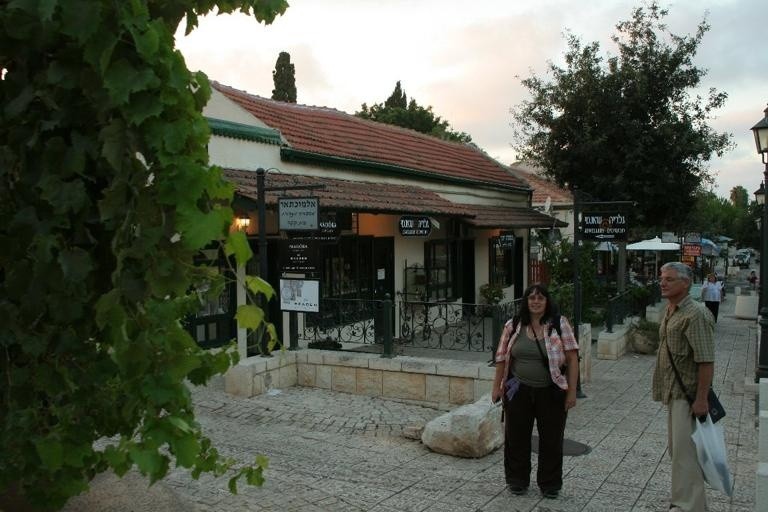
[478,283,507,317]
[627,318,662,355]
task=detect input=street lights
[750,101,768,378]
[236,213,250,358]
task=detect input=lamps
[238,211,251,232]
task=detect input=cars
[733,249,751,268]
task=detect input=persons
[701,273,724,322]
[491,285,580,498]
[652,261,715,512]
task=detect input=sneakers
[509,484,527,494]
[539,486,558,498]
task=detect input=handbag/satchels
[690,388,726,424]
